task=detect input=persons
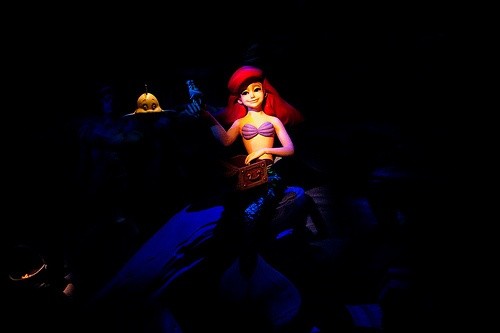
[188,65,307,229]
